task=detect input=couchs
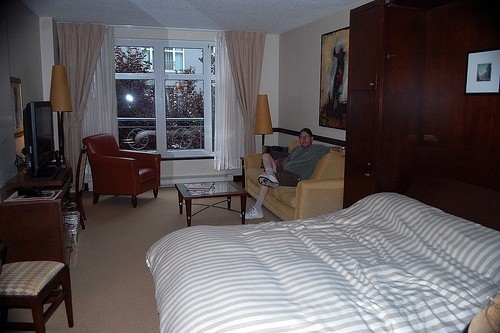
[245,139,345,222]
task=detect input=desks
[175,181,249,228]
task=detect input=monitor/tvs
[22,101,59,180]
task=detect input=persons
[240,128,346,219]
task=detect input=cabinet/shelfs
[0,166,75,304]
[344,0,423,209]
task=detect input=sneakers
[241,206,263,220]
[258,175,279,188]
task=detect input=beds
[144,192,500,333]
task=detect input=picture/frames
[465,50,500,95]
[319,26,351,130]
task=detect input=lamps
[254,95,273,154]
[49,65,71,157]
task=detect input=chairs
[66,144,88,230]
[0,243,74,333]
[82,133,161,208]
